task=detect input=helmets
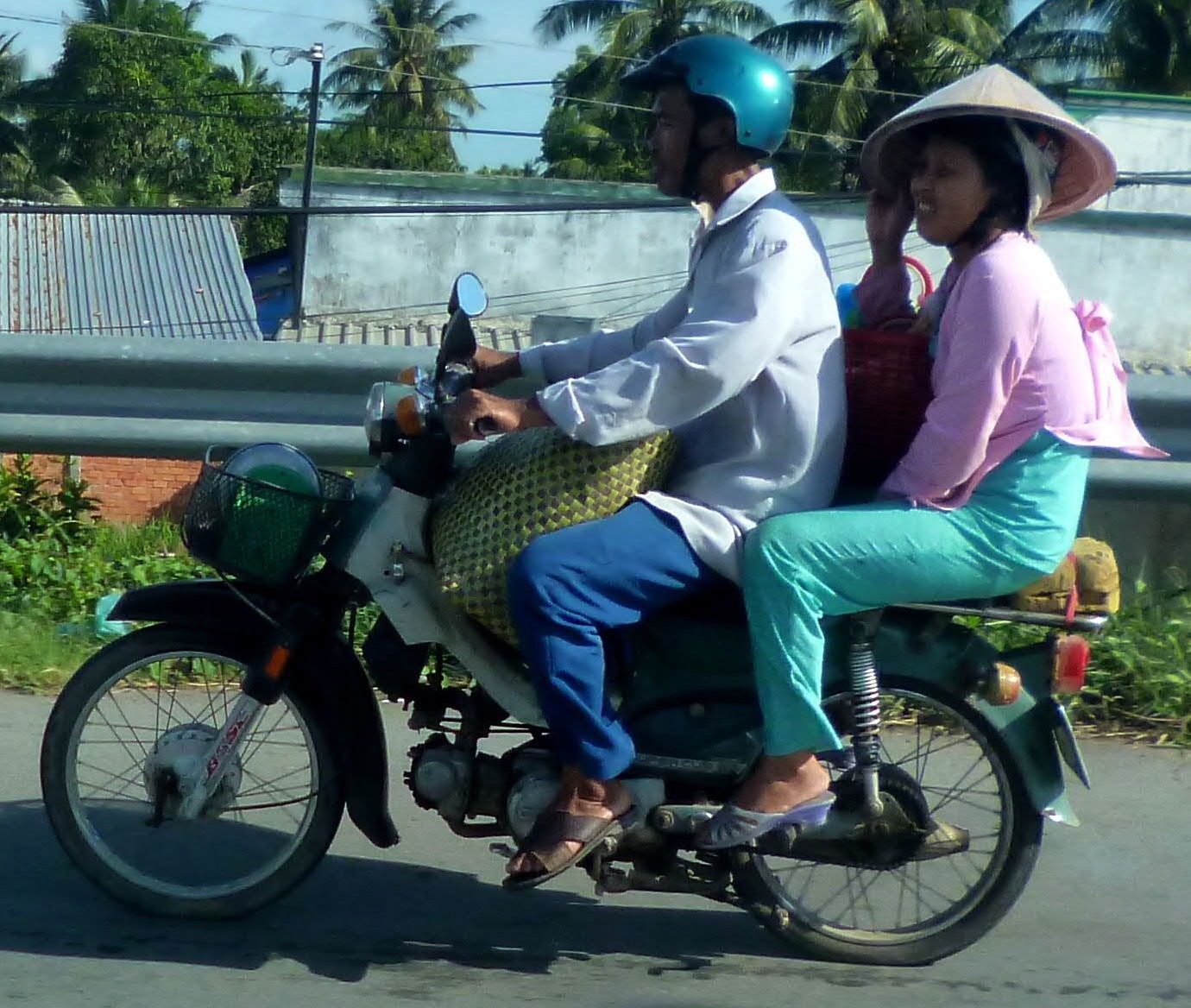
[620,34,795,161]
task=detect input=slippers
[691,789,836,850]
[499,799,645,889]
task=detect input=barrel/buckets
[207,440,324,577]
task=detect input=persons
[696,63,1115,850]
[441,34,838,892]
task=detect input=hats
[859,62,1118,224]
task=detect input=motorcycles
[36,267,1108,968]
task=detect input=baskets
[435,421,680,651]
